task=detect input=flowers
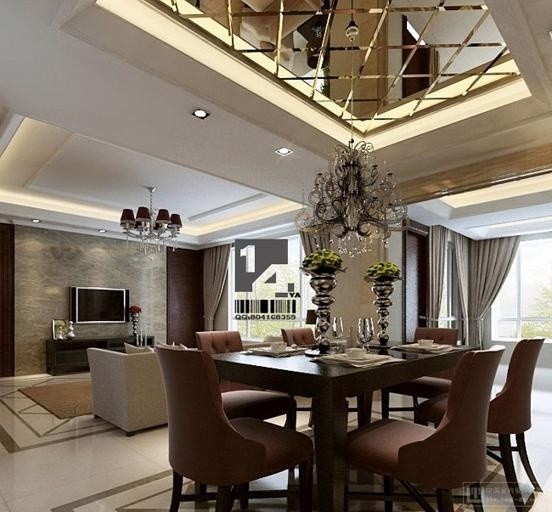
[364,262,401,276]
[129,305,141,313]
[303,249,344,268]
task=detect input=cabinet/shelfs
[45,336,154,376]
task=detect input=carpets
[17,380,93,419]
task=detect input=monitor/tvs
[69,286,129,324]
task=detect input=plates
[305,349,320,356]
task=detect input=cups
[345,348,367,358]
[269,342,286,351]
[419,340,433,347]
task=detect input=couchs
[87,347,168,436]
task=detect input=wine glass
[358,318,373,351]
[333,316,343,339]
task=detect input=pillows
[124,342,153,353]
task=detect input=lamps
[119,187,183,255]
[293,20,410,259]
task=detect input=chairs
[155,343,315,512]
[381,328,459,424]
[348,344,506,511]
[418,338,545,511]
[196,330,297,431]
[281,327,315,346]
